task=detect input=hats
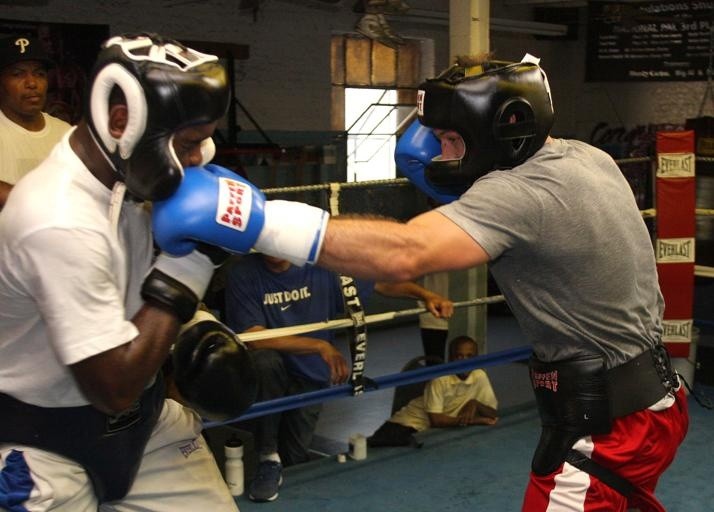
[0,31,55,70]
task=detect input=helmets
[78,27,231,204]
[412,50,558,199]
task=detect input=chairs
[392,356,449,419]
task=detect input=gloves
[165,308,265,428]
[138,239,234,324]
[147,162,332,272]
[388,114,462,203]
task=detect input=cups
[348,432,366,459]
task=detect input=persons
[368,335,499,447]
[223,246,454,503]
[151,52,691,512]
[0,34,253,512]
[2,34,72,202]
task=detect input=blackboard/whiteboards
[584,0,714,83]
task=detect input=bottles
[223,433,244,497]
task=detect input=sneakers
[247,454,285,503]
